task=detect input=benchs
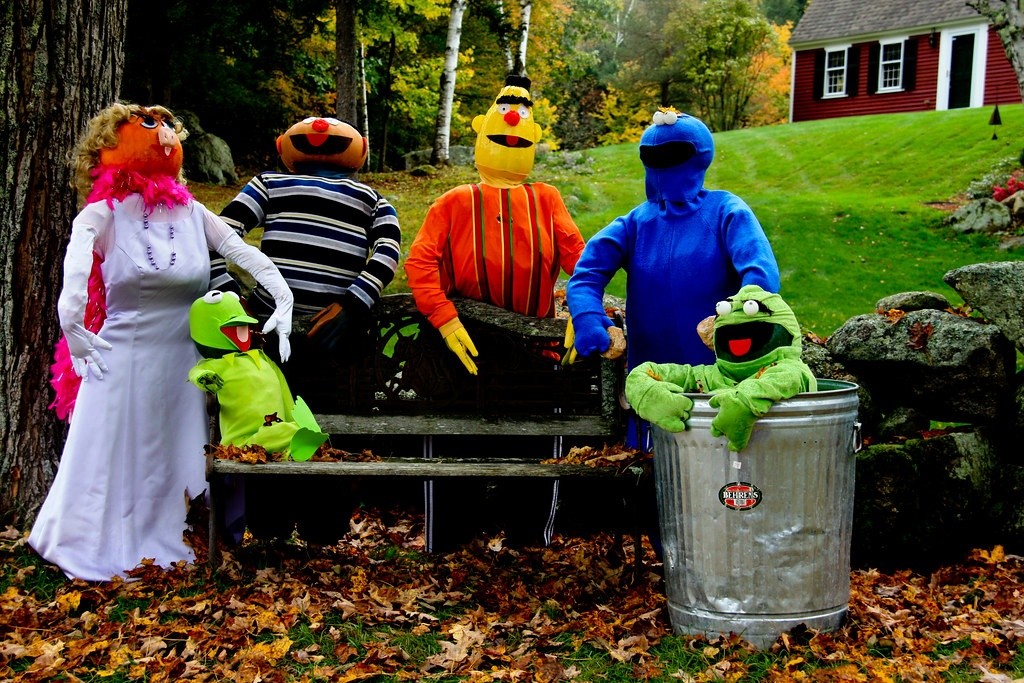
[205,294,657,555]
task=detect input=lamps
[928,34,937,47]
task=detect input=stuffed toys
[566,107,817,453]
[404,75,627,556]
[27,102,400,585]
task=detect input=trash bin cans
[652,378,862,653]
